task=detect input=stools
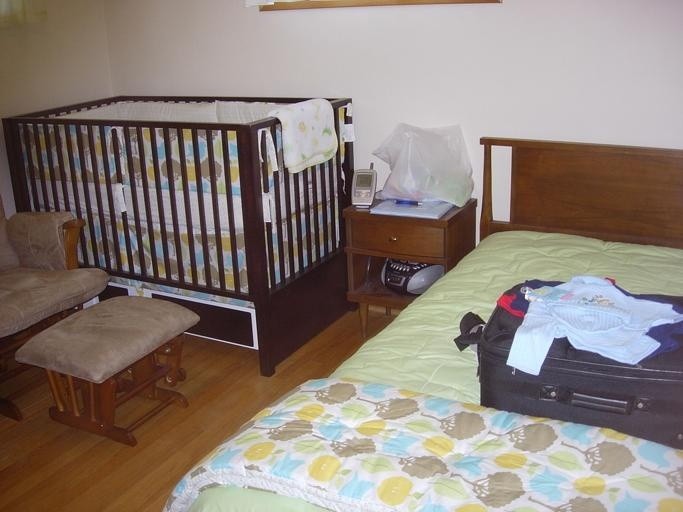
[13,297,202,447]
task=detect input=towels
[269,97,338,173]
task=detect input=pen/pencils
[394,200,424,205]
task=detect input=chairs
[0,195,110,424]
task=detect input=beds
[4,90,359,380]
[157,137,683,510]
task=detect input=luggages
[453,281,683,452]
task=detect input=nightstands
[343,196,479,338]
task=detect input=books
[370,199,454,220]
